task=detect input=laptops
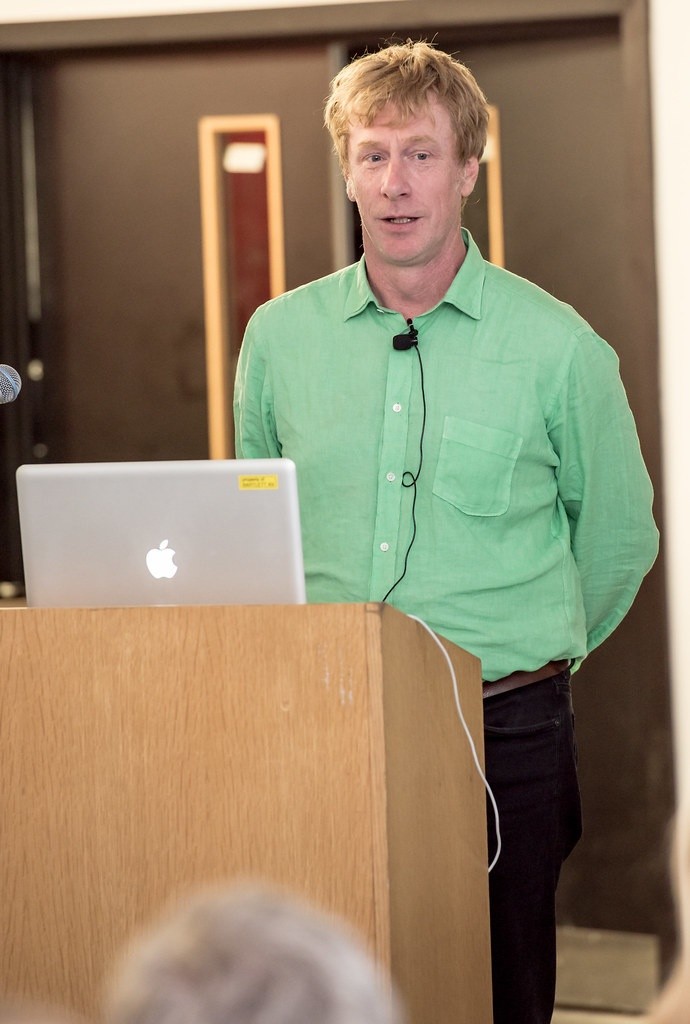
[15,457,308,610]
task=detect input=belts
[483,659,568,699]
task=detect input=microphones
[393,334,418,351]
[0,364,22,406]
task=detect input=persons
[103,878,400,1024]
[232,40,661,1024]
